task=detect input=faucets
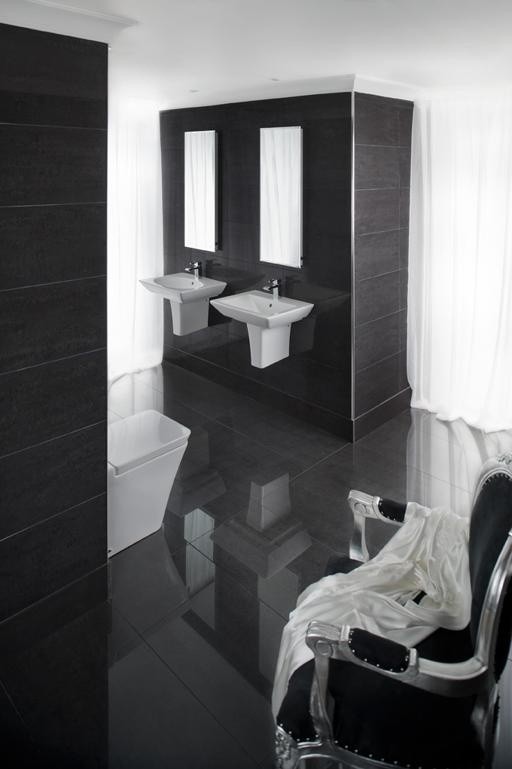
[263,277,280,299]
[184,261,203,279]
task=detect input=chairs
[273,453,511,769]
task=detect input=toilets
[106,410,192,560]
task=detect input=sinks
[139,271,227,301]
[210,289,304,328]
